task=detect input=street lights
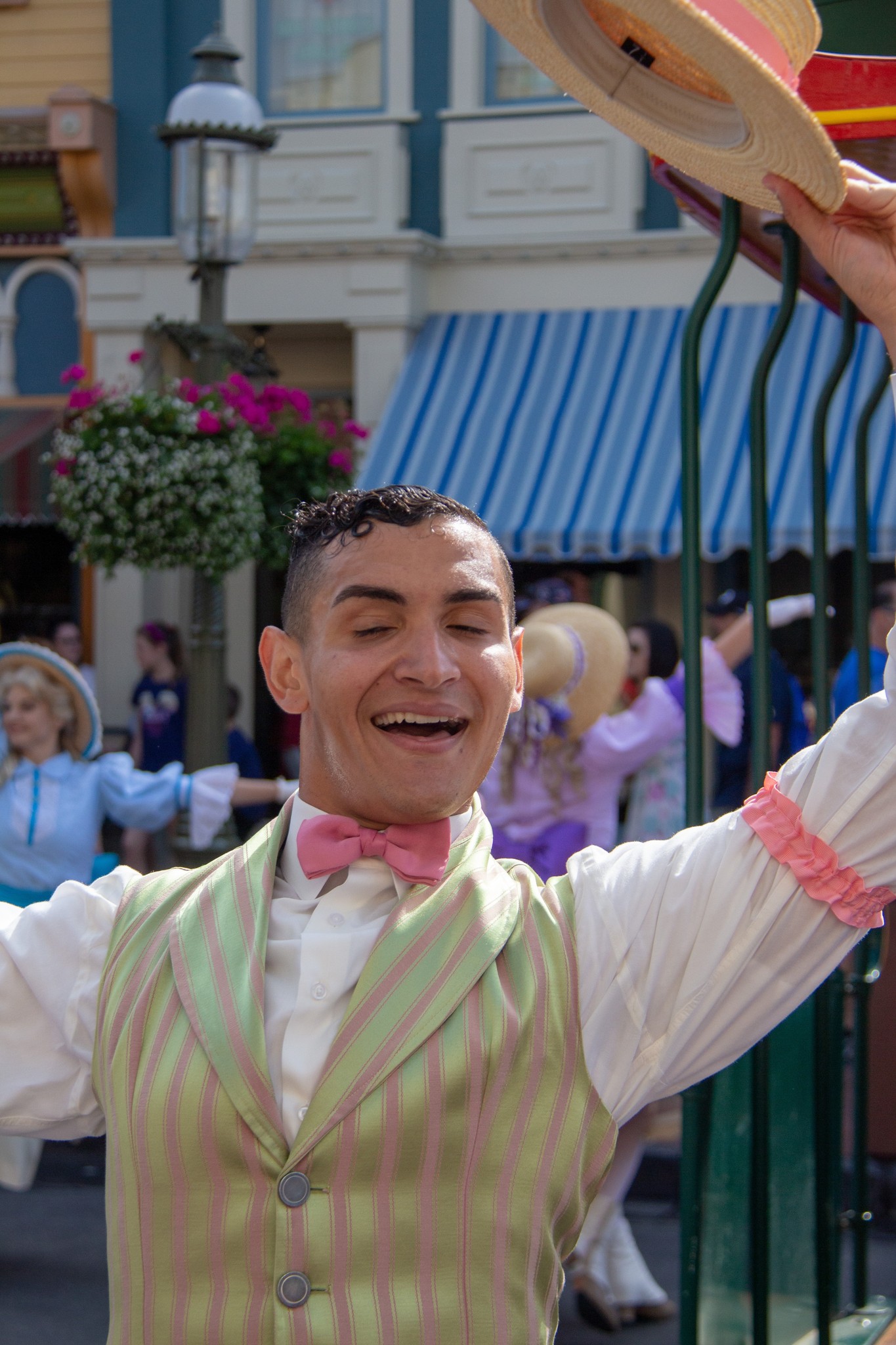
[154,32,282,773]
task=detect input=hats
[706,585,750,617]
[0,641,104,762]
[466,0,848,215]
[503,602,633,778]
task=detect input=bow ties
[296,814,453,888]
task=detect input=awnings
[347,311,896,565]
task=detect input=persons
[0,160,896,1345]
[0,642,299,1194]
[481,586,896,1330]
[51,622,269,876]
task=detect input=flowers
[42,349,370,576]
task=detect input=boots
[569,1195,677,1332]
